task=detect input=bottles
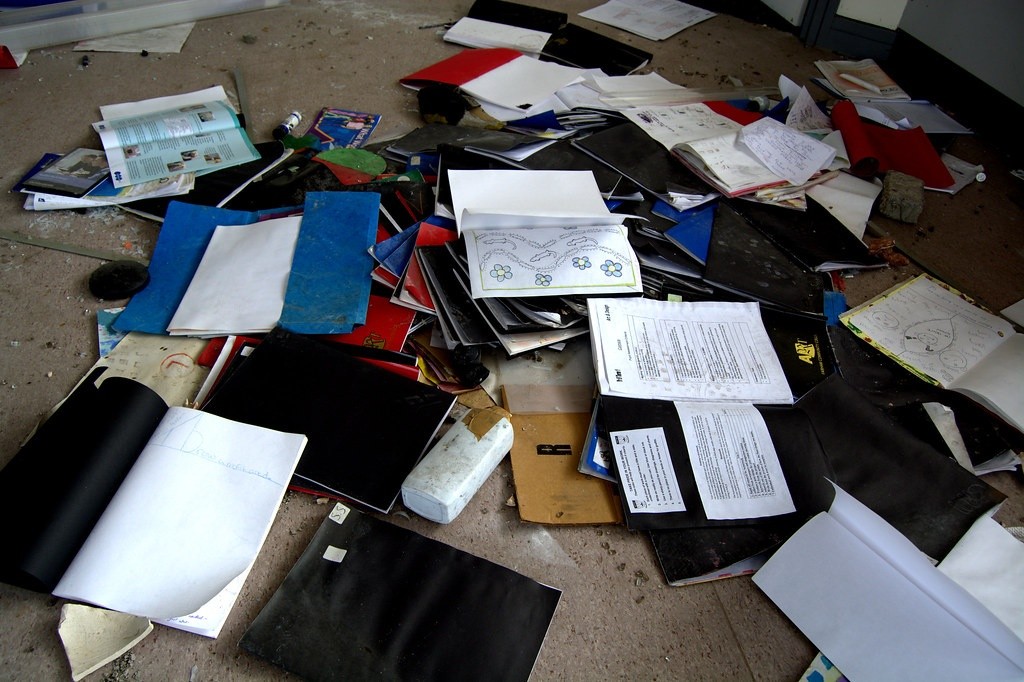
[748,96,770,112]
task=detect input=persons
[58,153,109,180]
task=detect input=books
[0,0,1024,682]
